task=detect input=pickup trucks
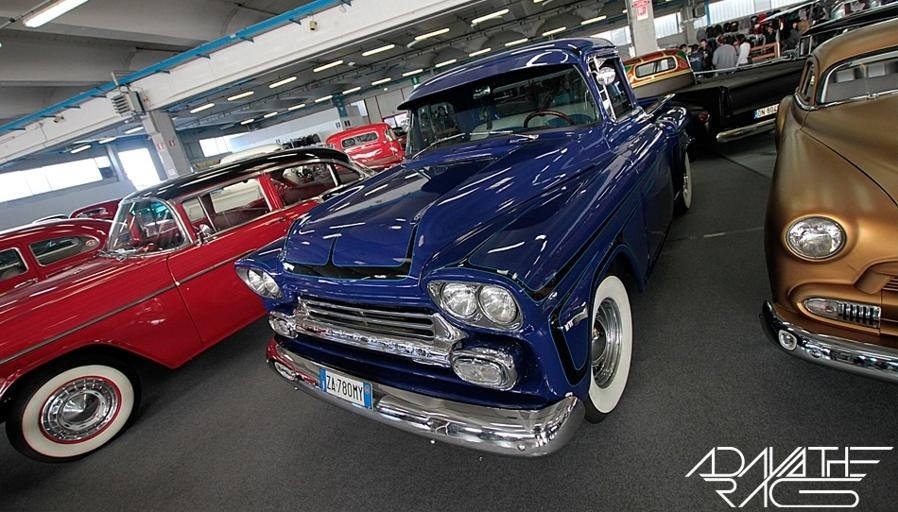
[618,48,810,148]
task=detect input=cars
[233,37,695,457]
[220,143,290,165]
[758,0,819,44]
[392,127,407,146]
[795,0,898,59]
[762,17,897,386]
[31,214,68,222]
[0,142,379,467]
[0,218,184,286]
[69,198,122,218]
[324,124,404,170]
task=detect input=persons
[435,105,455,132]
[675,7,809,73]
